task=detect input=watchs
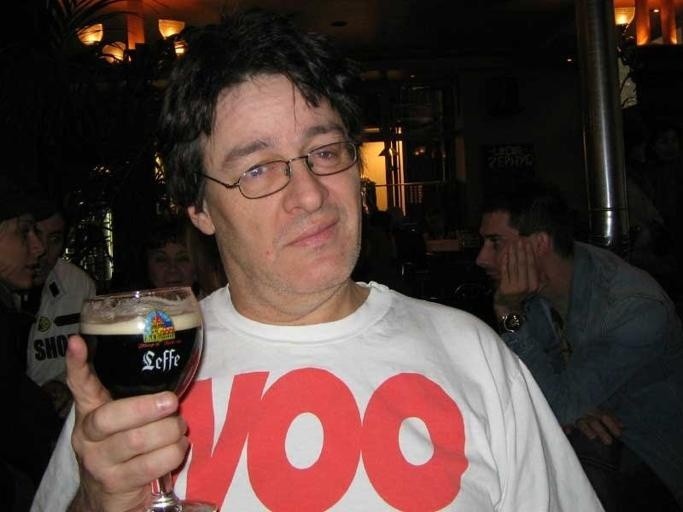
[495,313,525,333]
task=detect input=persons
[187,237,227,300]
[30,10,601,512]
[0,181,75,512]
[25,191,98,419]
[140,212,200,300]
[472,178,683,512]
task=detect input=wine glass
[79,286,217,512]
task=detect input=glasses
[201,140,358,199]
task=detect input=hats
[0,176,57,223]
